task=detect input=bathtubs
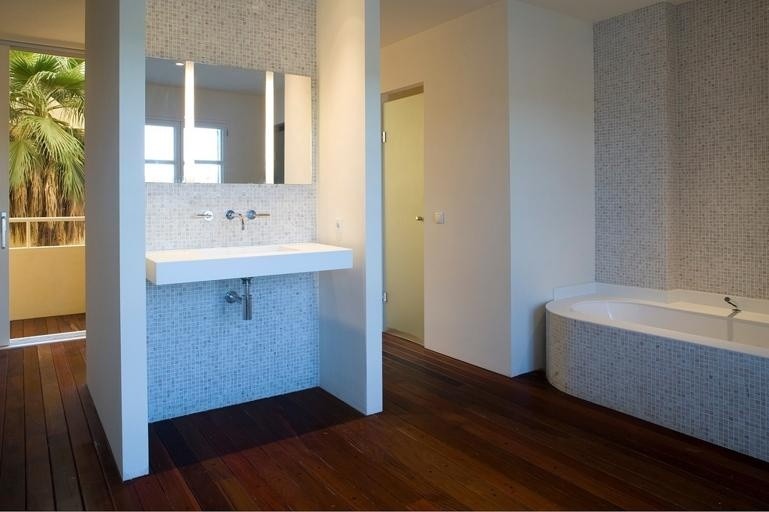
[544,294,769,463]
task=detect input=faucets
[226,210,245,230]
[723,297,742,312]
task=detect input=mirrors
[145,56,313,185]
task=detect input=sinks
[145,242,354,287]
